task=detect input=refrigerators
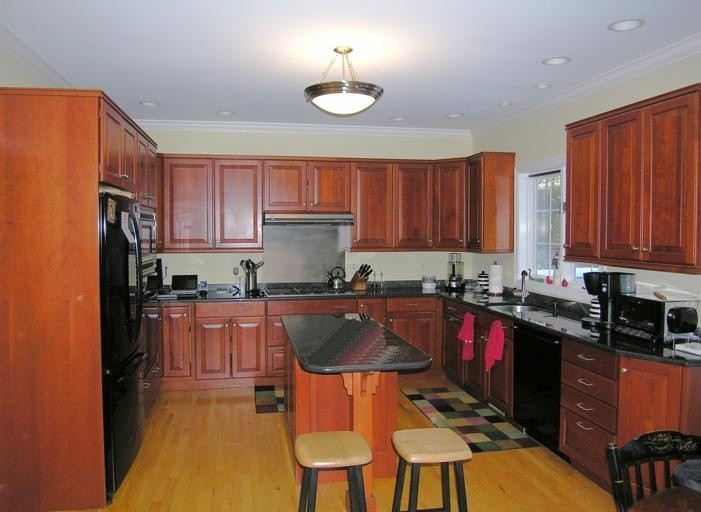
[100,192,144,505]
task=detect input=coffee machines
[582,272,636,329]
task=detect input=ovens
[142,259,162,412]
[513,313,587,450]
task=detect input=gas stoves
[262,288,356,297]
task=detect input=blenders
[444,253,464,292]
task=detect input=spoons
[240,259,264,272]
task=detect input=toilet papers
[487,263,505,294]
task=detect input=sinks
[486,301,541,313]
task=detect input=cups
[245,273,257,292]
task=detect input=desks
[278,313,433,484]
[626,484,701,512]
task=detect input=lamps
[304,47,383,116]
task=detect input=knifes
[358,263,372,277]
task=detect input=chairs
[605,431,701,512]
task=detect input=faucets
[518,269,530,302]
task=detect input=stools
[294,430,370,511]
[389,428,473,511]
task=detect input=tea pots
[325,267,346,289]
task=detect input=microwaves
[140,210,157,265]
[611,291,701,349]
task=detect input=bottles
[477,271,488,293]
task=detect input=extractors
[264,214,354,227]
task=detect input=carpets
[254,385,285,414]
[401,388,541,454]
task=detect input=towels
[484,321,505,372]
[457,311,475,361]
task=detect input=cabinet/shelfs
[163,301,192,392]
[443,301,465,391]
[158,153,263,253]
[192,301,267,393]
[343,157,394,252]
[600,82,701,275]
[394,159,433,251]
[466,151,515,254]
[563,112,601,264]
[433,157,466,251]
[558,336,701,496]
[267,298,358,387]
[466,305,517,425]
[386,296,443,381]
[263,156,351,213]
[357,298,386,327]
[138,125,158,212]
[0,86,137,192]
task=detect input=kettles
[421,273,437,288]
